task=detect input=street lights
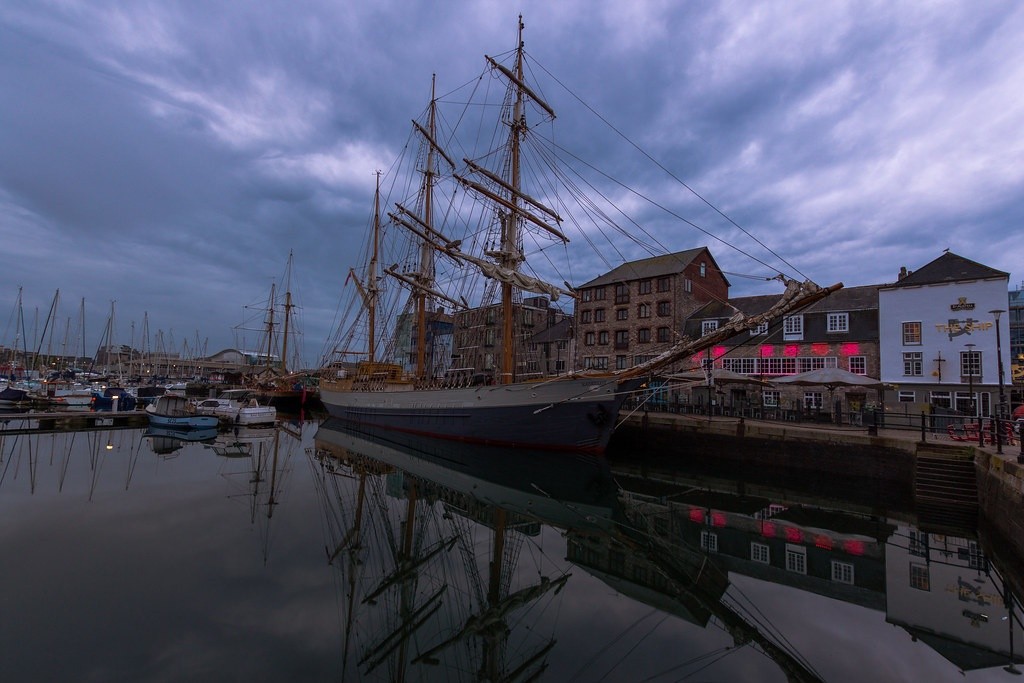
[965,343,978,424]
[988,308,1009,435]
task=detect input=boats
[199,427,278,459]
[142,423,219,477]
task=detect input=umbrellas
[667,369,775,415]
[766,368,889,424]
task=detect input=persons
[486,375,496,386]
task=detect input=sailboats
[217,412,324,566]
[0,247,316,429]
[304,417,833,683]
[317,14,845,455]
